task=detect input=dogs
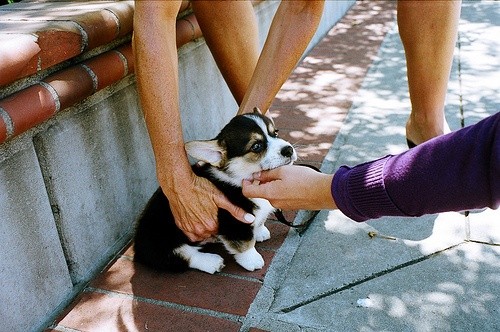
[132,107,302,274]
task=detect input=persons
[133,0,463,243]
[242,111,500,224]
